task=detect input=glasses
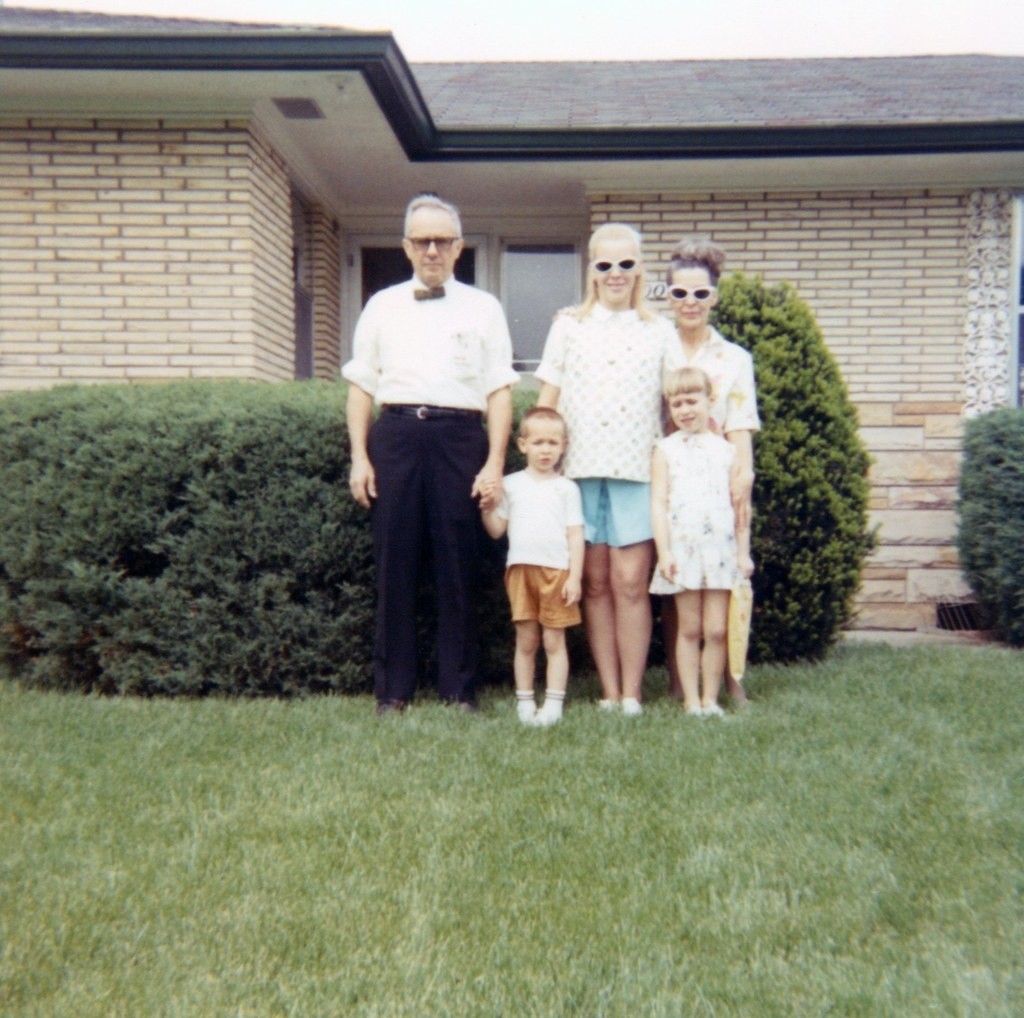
[668,284,716,302]
[407,235,458,252]
[591,258,637,274]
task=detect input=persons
[652,367,754,718]
[340,195,520,715]
[535,221,685,717]
[480,408,586,726]
[668,241,761,708]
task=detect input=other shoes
[446,699,476,713]
[703,704,724,718]
[623,698,643,718]
[516,701,537,725]
[375,697,405,717]
[535,700,564,728]
[599,699,619,712]
[687,706,701,716]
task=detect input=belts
[383,404,480,419]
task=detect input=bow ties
[414,286,445,301]
